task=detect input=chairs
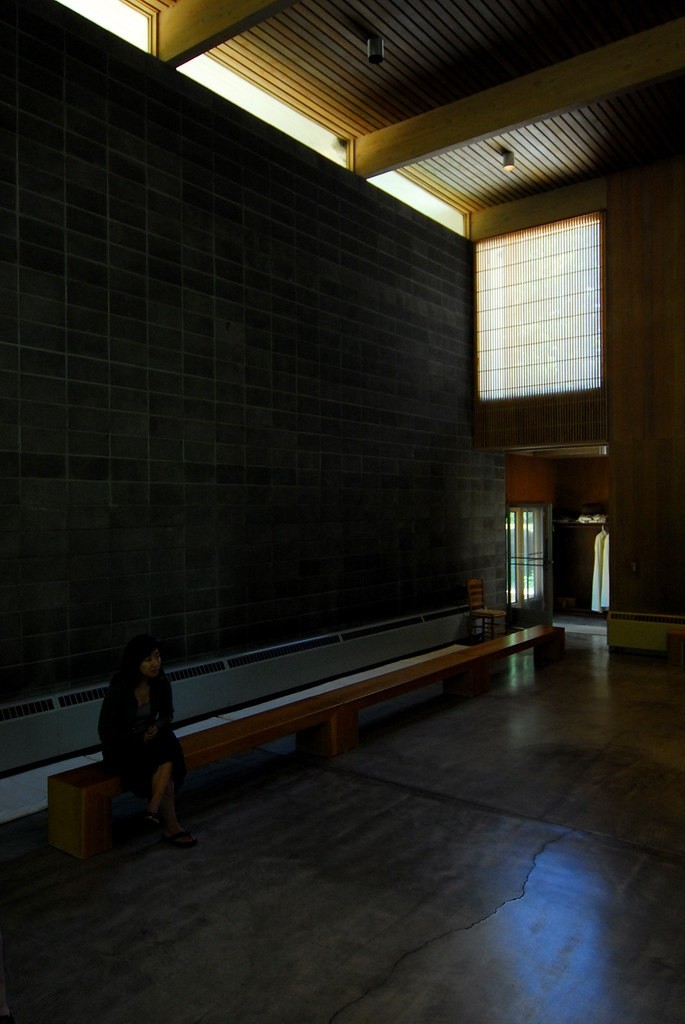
[468,579,508,640]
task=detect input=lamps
[367,37,384,64]
[501,150,513,166]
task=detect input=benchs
[666,629,685,667]
[48,624,564,861]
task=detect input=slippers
[142,812,161,826]
[161,831,197,849]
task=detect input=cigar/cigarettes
[145,735,153,736]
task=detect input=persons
[98,632,198,850]
